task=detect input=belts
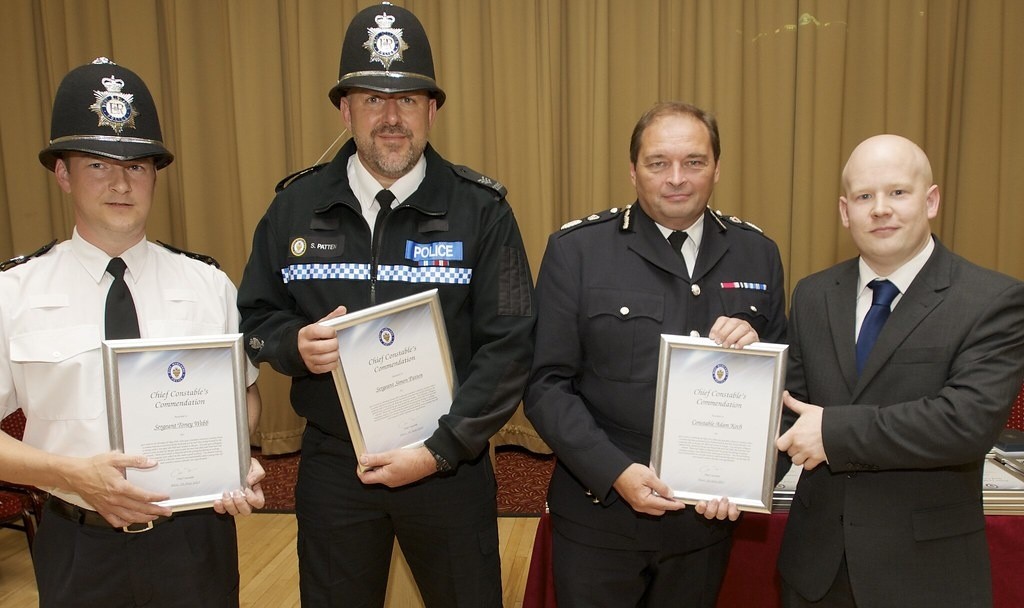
[49,496,174,531]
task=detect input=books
[979,446,1022,516]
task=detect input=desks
[523,431,1022,607]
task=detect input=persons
[0,55,272,607]
[688,130,1024,608]
[525,99,789,608]
[234,0,531,608]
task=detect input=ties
[854,280,902,381]
[372,189,397,254]
[101,255,140,340]
[667,231,688,266]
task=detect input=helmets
[37,55,173,176]
[328,5,445,111]
